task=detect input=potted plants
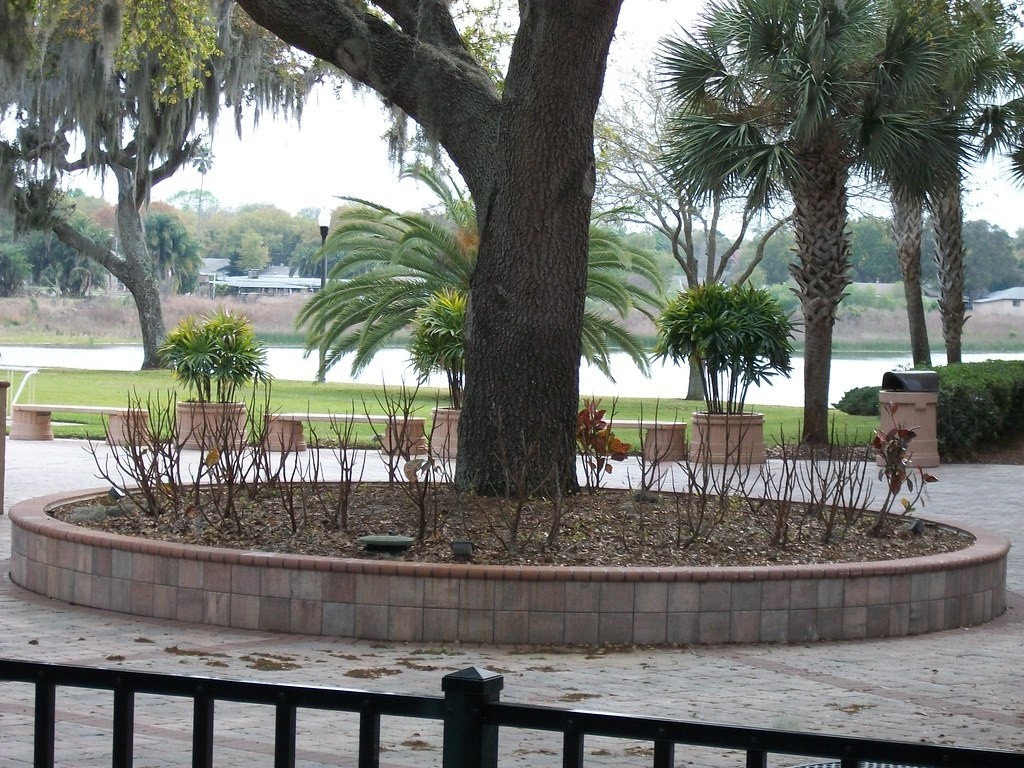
[647,276,806,464]
[154,303,275,452]
[402,286,470,459]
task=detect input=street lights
[318,209,331,378]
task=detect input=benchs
[8,403,149,446]
[261,412,428,456]
[600,419,687,462]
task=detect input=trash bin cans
[874,371,941,468]
